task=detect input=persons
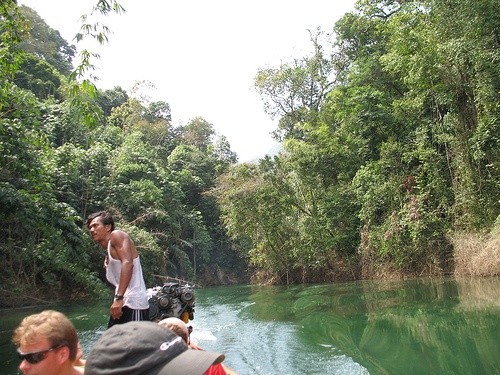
[11,309,85,375]
[86,211,150,328]
[83,319,226,375]
[158,317,225,375]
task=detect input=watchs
[113,294,123,300]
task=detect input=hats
[84,321,225,375]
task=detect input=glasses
[17,343,65,364]
